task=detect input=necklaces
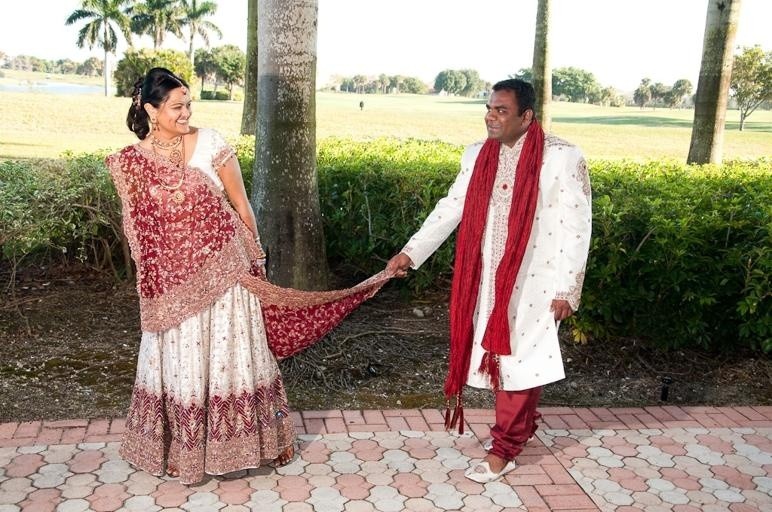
[148,128,187,189]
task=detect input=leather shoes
[484,433,534,452]
[464,458,516,485]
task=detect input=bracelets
[254,236,269,265]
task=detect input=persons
[383,78,593,484]
[104,67,395,486]
